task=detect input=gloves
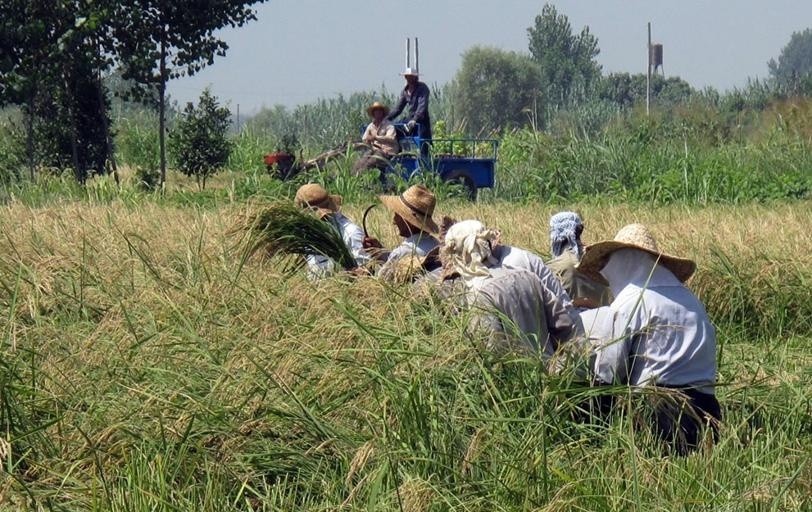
[407,120,417,129]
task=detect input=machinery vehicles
[262,122,504,204]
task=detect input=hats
[376,183,442,235]
[575,222,697,284]
[293,183,344,220]
[366,101,390,117]
[398,67,425,77]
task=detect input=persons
[364,183,721,449]
[294,183,371,279]
[352,100,399,176]
[387,67,433,152]
